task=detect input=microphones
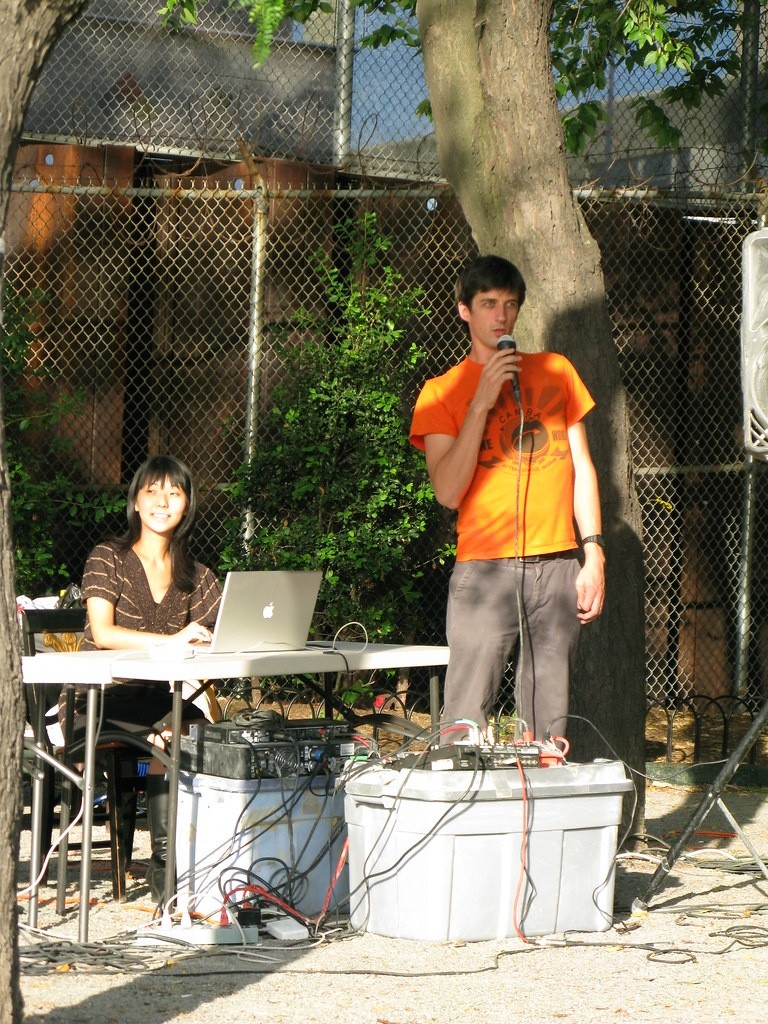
[496,333,522,406]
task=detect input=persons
[409,255,604,749]
[59,455,223,904]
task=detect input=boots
[146,776,179,906]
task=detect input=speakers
[740,228,768,462]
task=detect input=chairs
[19,607,149,867]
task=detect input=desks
[35,636,449,919]
[19,653,112,944]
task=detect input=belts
[519,550,580,563]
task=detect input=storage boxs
[162,752,635,938]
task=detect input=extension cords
[137,927,260,946]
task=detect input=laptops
[181,569,323,654]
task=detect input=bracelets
[582,534,604,546]
